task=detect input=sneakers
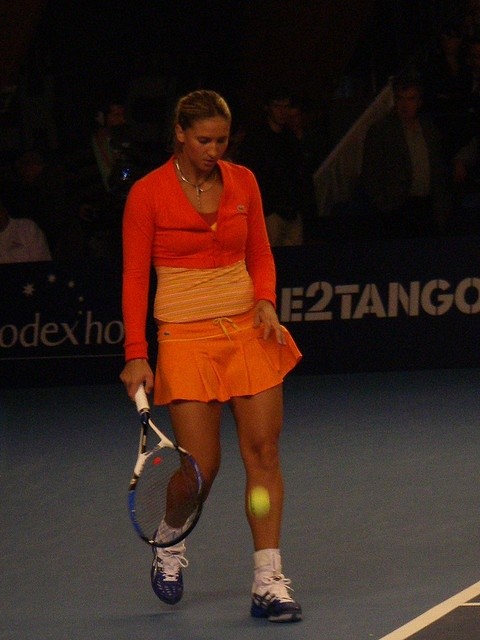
[147,528,190,603]
[249,570,303,623]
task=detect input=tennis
[249,486,271,517]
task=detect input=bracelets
[175,158,218,210]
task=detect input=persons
[0,93,131,270]
[119,88,302,622]
[263,2,479,244]
